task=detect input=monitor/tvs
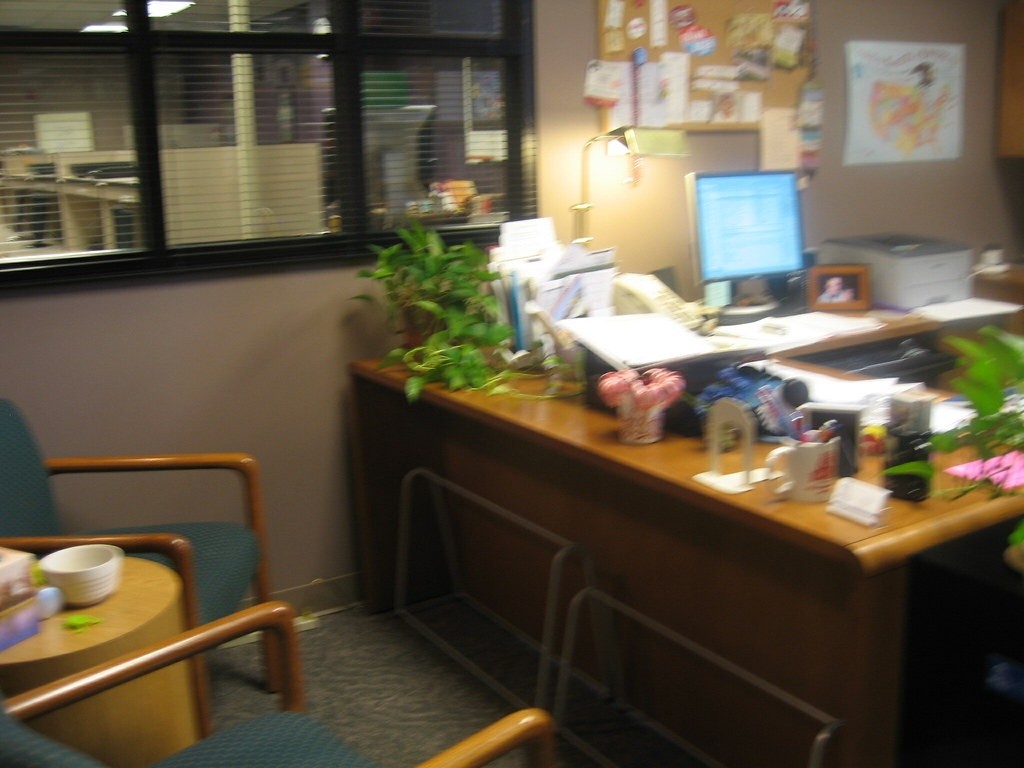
[685,169,806,314]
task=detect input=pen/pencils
[755,385,840,444]
[487,266,597,353]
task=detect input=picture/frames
[806,264,871,310]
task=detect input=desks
[693,257,1024,393]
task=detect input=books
[557,308,768,374]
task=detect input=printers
[816,232,977,310]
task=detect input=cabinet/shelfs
[350,355,1024,768]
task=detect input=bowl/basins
[38,544,124,609]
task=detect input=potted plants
[348,217,587,403]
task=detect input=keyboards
[818,343,929,373]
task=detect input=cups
[883,430,933,502]
[765,435,843,503]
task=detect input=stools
[0,556,198,768]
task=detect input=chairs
[0,601,555,768]
[0,397,278,740]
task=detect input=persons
[816,276,856,302]
